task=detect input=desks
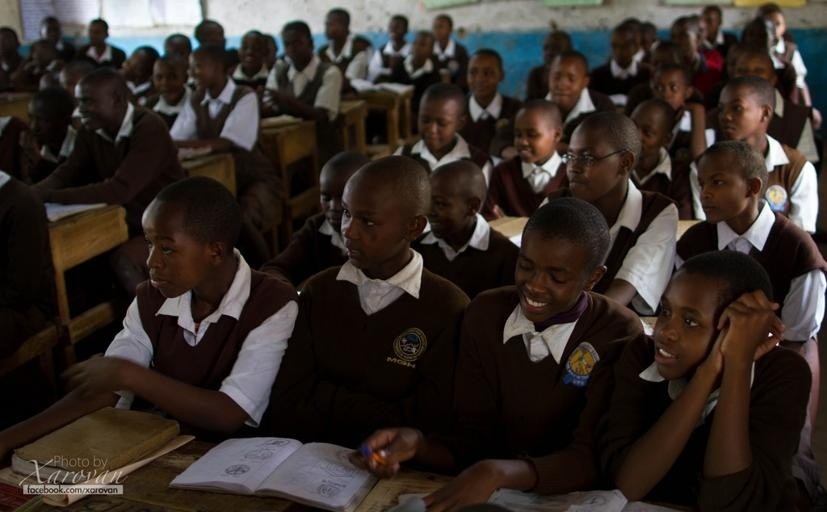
[44,200,129,345]
[641,314,659,341]
[0,91,38,129]
[367,83,415,154]
[261,111,319,232]
[177,148,237,252]
[0,439,711,511]
[335,101,367,152]
[488,215,707,253]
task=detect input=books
[0,433,200,505]
[167,438,373,510]
[403,482,667,511]
[13,406,180,485]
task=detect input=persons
[170,42,261,207]
[488,99,567,217]
[528,31,573,104]
[117,47,160,108]
[259,152,372,289]
[194,19,241,70]
[164,34,192,76]
[261,32,280,66]
[640,22,666,67]
[18,90,92,187]
[585,22,653,116]
[536,110,679,316]
[387,28,441,98]
[225,29,270,92]
[255,155,475,449]
[432,14,471,80]
[31,72,184,315]
[0,175,300,439]
[73,19,126,70]
[690,74,819,234]
[410,158,521,298]
[755,2,822,125]
[372,15,414,84]
[349,198,644,511]
[112,53,195,149]
[18,38,67,87]
[649,64,715,162]
[315,7,372,89]
[1,27,32,88]
[458,48,522,161]
[388,80,495,191]
[259,19,343,153]
[701,5,738,64]
[733,52,818,165]
[647,37,692,74]
[673,16,725,92]
[671,139,827,350]
[37,16,76,60]
[608,251,813,511]
[740,16,799,106]
[526,51,616,143]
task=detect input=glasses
[562,148,620,167]
